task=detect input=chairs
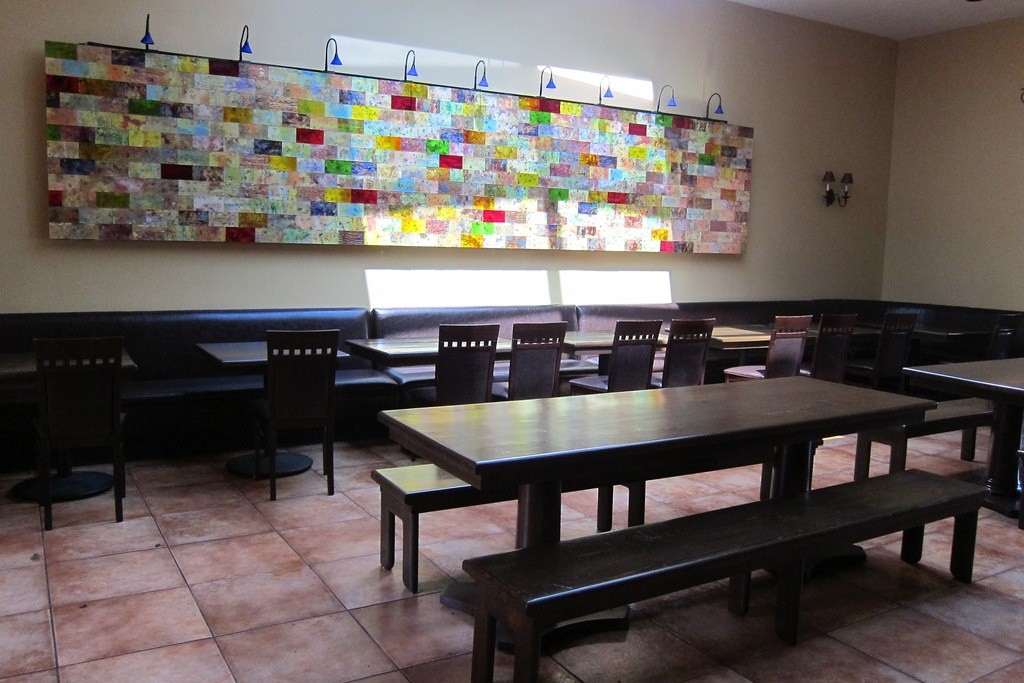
[251,329,340,501]
[569,319,663,395]
[723,314,813,384]
[650,317,717,390]
[404,326,499,461]
[985,312,1022,361]
[798,314,858,384]
[846,312,918,395]
[491,322,568,401]
[27,334,129,532]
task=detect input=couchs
[0,309,403,476]
[575,305,759,372]
[371,304,579,443]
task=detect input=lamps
[657,85,676,113]
[238,25,252,61]
[821,171,854,207]
[598,76,613,105]
[706,93,724,119]
[539,66,556,98]
[474,61,489,91]
[141,14,156,49]
[403,50,418,82]
[325,38,342,72]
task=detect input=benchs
[462,468,992,683]
[854,393,993,483]
[369,438,826,594]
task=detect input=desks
[0,346,139,505]
[377,376,939,657]
[344,320,1023,457]
[903,357,1024,519]
[194,340,350,479]
